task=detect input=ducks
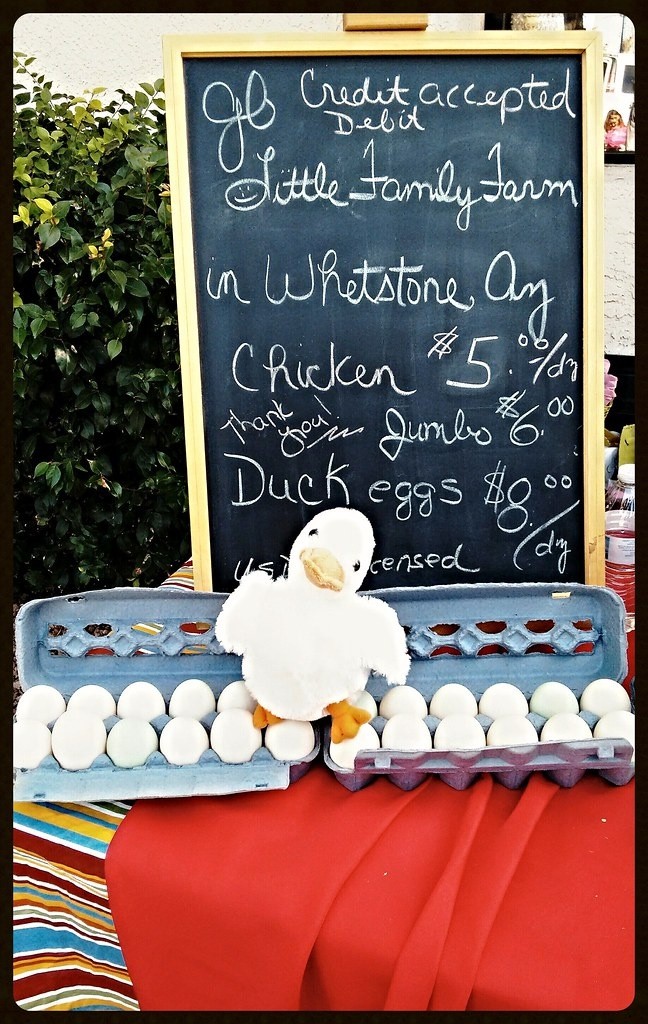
[216,506,412,739]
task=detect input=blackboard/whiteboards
[161,32,604,596]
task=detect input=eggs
[13,679,635,770]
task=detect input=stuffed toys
[213,505,412,745]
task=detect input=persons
[605,110,628,150]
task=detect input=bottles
[606,463,635,633]
